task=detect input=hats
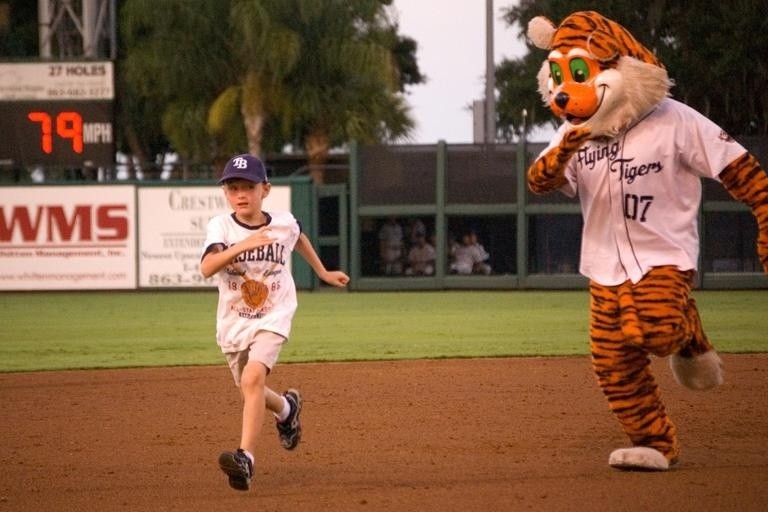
[217,155,269,185]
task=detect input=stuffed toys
[521,9,768,471]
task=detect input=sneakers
[218,448,254,491]
[275,387,302,451]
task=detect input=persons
[196,150,352,491]
[373,216,495,275]
